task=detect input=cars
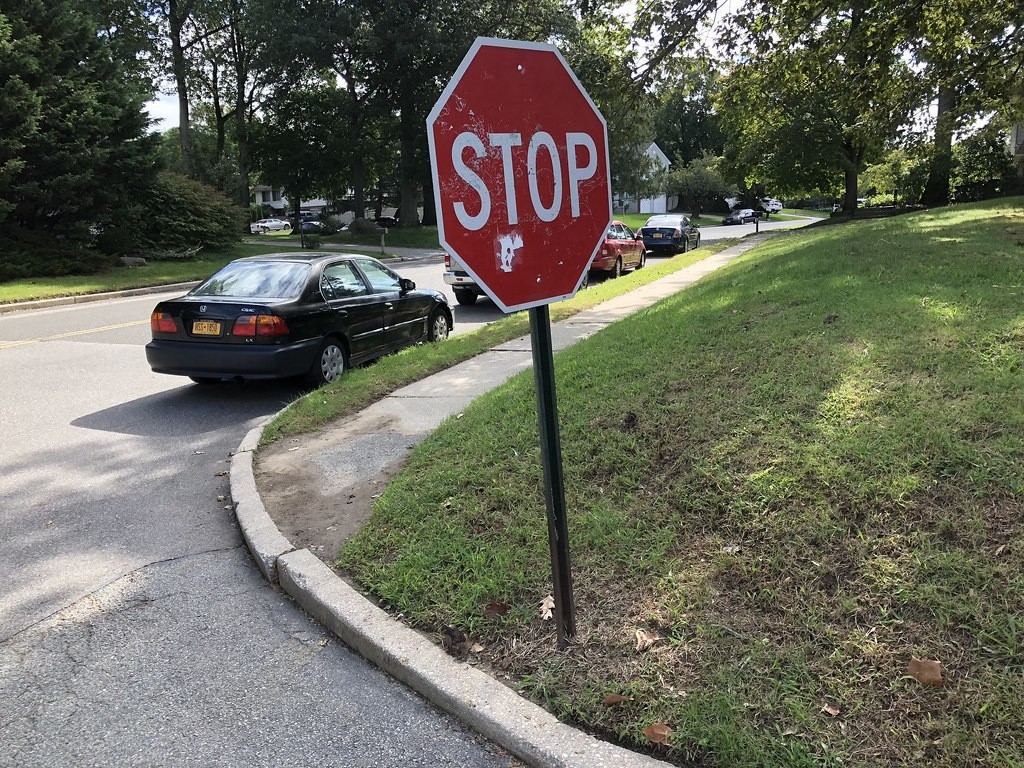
[251,219,292,233]
[589,220,647,278]
[755,196,783,214]
[634,214,701,253]
[371,216,398,229]
[146,251,457,389]
[722,209,758,225]
[443,248,589,305]
[289,211,315,222]
[301,221,324,230]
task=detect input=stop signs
[425,35,615,316]
